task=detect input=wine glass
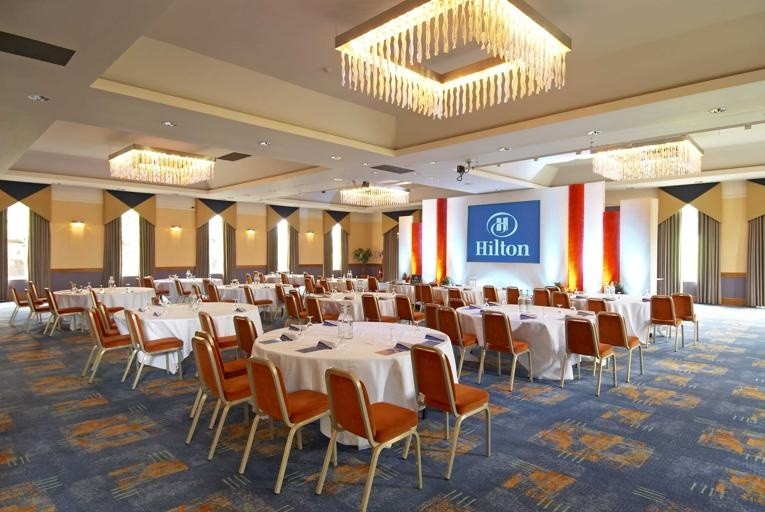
[448,280,452,287]
[483,298,490,307]
[306,315,314,330]
[232,298,240,313]
[573,288,579,297]
[124,284,131,294]
[556,304,563,315]
[86,281,91,289]
[391,288,396,296]
[413,321,422,337]
[79,286,84,294]
[98,284,104,294]
[333,286,338,294]
[617,292,622,301]
[569,302,577,313]
[501,296,508,305]
[330,289,335,298]
[196,294,203,303]
[374,290,381,300]
[644,289,650,297]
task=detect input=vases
[360,263,365,276]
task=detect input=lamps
[335,0,571,120]
[591,137,704,183]
[340,185,408,208]
[109,144,215,186]
[172,225,181,233]
[307,231,314,236]
[70,219,84,226]
[246,228,256,234]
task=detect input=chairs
[189,330,249,428]
[23,289,51,333]
[592,312,643,383]
[476,310,532,391]
[645,295,684,352]
[436,306,484,380]
[185,338,252,461]
[559,317,616,397]
[665,293,699,347]
[120,311,184,390]
[238,356,338,494]
[199,312,238,359]
[27,281,46,300]
[315,368,423,512]
[42,288,85,337]
[81,304,132,384]
[9,288,41,324]
[402,344,492,480]
[232,316,258,354]
[91,291,124,315]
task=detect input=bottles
[356,279,364,297]
[349,270,352,279]
[347,270,349,279]
[524,290,532,312]
[108,276,116,290]
[185,269,192,280]
[253,272,260,286]
[517,290,524,313]
[610,280,615,297]
[188,289,198,307]
[469,279,476,289]
[604,281,610,298]
[337,303,345,338]
[343,304,354,339]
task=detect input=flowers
[353,247,373,263]
